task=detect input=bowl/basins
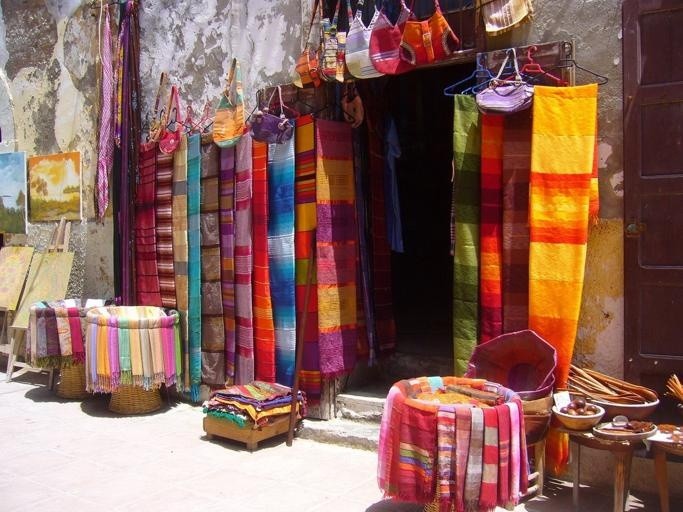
[551,401,607,431]
[586,394,662,422]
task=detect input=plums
[560,398,597,415]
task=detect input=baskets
[86,303,179,416]
[28,298,106,400]
[389,377,528,512]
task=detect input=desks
[566,431,683,510]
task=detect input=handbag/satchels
[476,85,534,116]
[251,110,293,145]
[158,130,181,155]
[398,11,462,67]
[148,121,165,142]
[340,96,364,130]
[291,48,323,89]
[211,94,246,149]
[319,21,353,86]
[368,9,426,76]
[344,14,393,81]
[479,1,534,38]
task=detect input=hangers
[156,106,213,140]
[244,83,356,124]
[441,42,608,97]
